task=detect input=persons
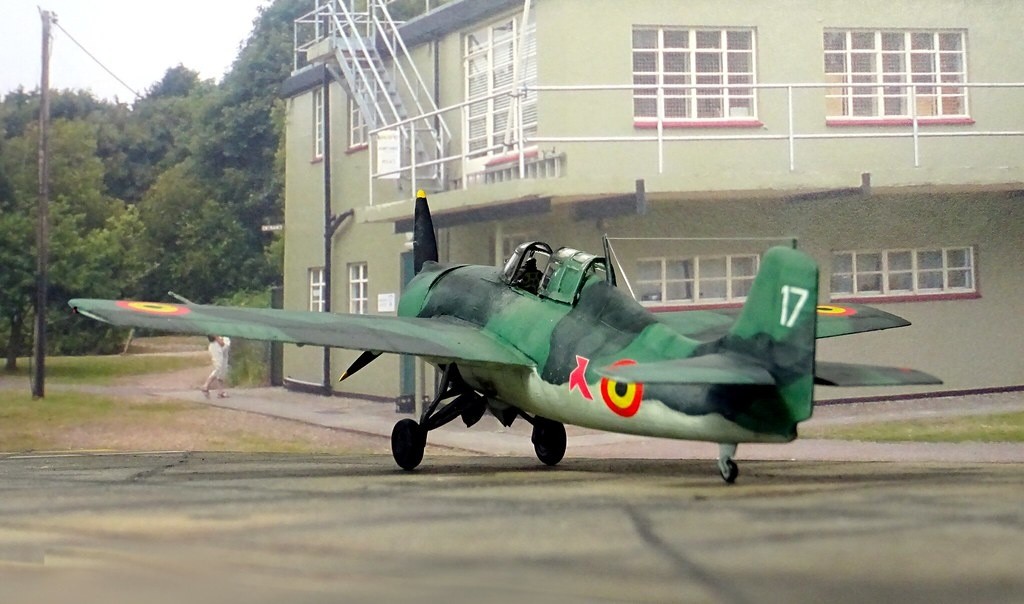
[199,334,232,400]
[514,257,544,297]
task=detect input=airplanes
[60,189,946,486]
